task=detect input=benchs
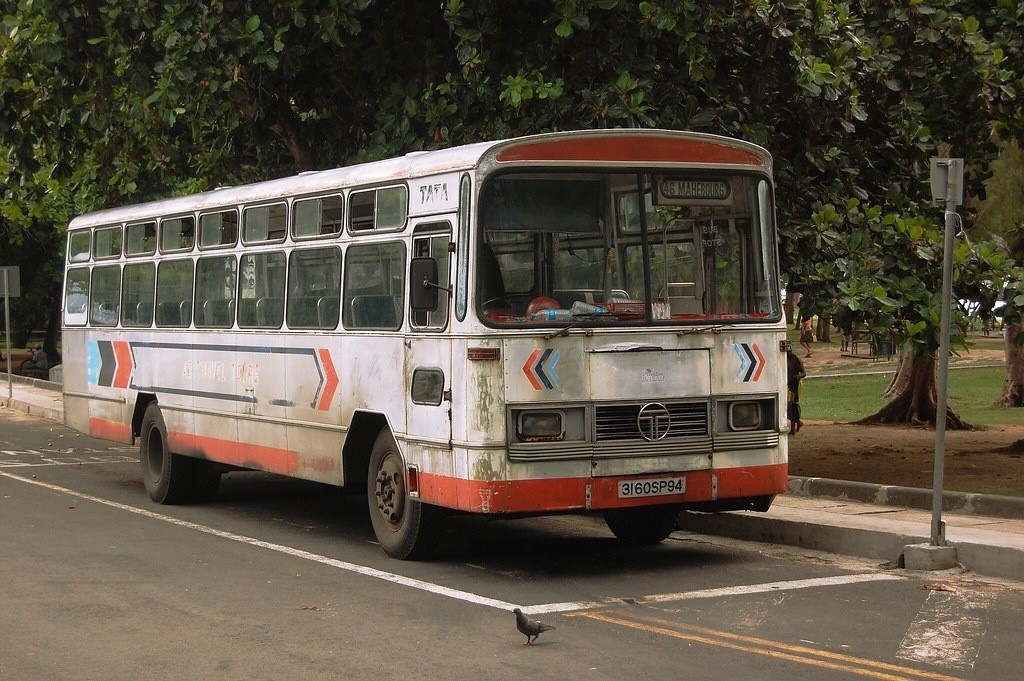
[81,290,630,328]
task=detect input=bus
[58,125,795,563]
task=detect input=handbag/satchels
[813,334,818,343]
[791,404,801,421]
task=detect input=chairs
[482,242,506,303]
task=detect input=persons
[840,328,851,351]
[787,349,806,435]
[981,317,990,336]
[27,343,49,370]
[800,316,814,358]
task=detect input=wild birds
[511,607,554,645]
